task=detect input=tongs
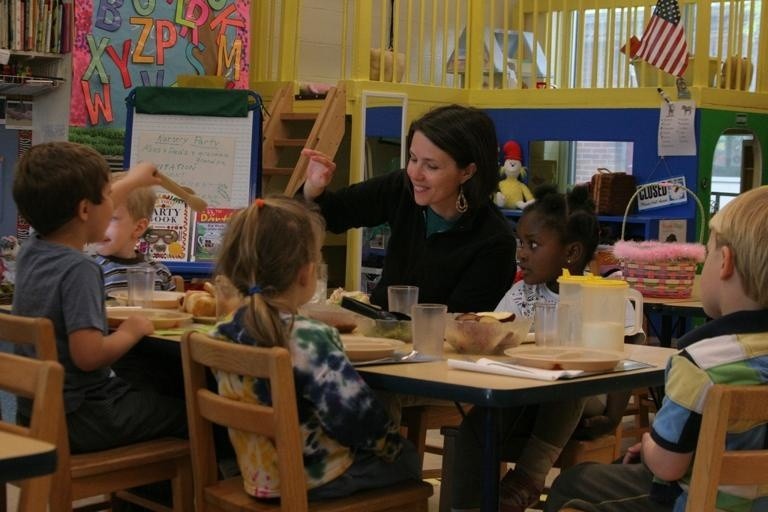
[152,173,209,214]
[340,295,411,326]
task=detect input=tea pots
[556,267,644,349]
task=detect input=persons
[89,170,184,394]
[438,182,647,512]
[202,196,427,507]
[542,182,768,511]
[8,139,193,508]
[290,103,518,511]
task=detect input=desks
[641,293,712,348]
[1,430,58,512]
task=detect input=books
[0,0,76,58]
[190,205,248,264]
[134,190,188,262]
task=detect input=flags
[634,0,691,79]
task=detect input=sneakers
[492,468,541,511]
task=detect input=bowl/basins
[437,318,531,354]
[299,303,357,333]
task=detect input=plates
[108,289,187,308]
[504,344,618,375]
[339,336,400,360]
[104,309,193,329]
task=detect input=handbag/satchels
[591,168,635,216]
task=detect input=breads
[455,311,516,354]
[184,281,226,317]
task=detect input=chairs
[2,313,194,510]
[505,279,644,512]
[181,330,435,510]
[685,382,768,511]
[1,354,72,512]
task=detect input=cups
[409,303,448,356]
[529,298,570,350]
[215,286,244,325]
[123,265,159,311]
[389,285,419,315]
